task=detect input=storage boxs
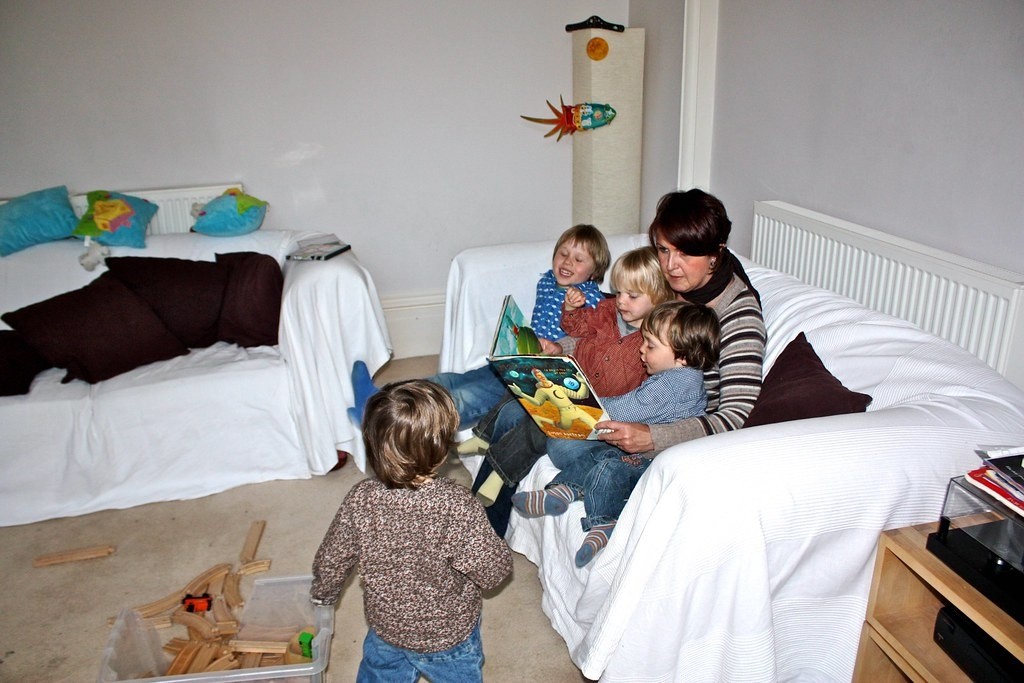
[95,573,335,683]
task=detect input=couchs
[438,233,1024,683]
[0,227,394,527]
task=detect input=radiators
[748,199,1024,391]
[0,182,244,235]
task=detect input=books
[285,233,351,261]
[964,453,1024,517]
[485,295,615,440]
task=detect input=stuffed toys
[77,240,111,272]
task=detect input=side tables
[851,511,1024,683]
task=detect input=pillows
[0,271,192,384]
[213,251,283,349]
[743,331,872,428]
[0,331,45,396]
[103,256,227,349]
[0,184,81,258]
[70,191,159,248]
[191,192,272,237]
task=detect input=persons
[309,379,514,683]
[347,224,611,433]
[511,300,721,568]
[449,245,677,507]
[471,187,768,541]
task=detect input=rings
[616,441,620,447]
[570,290,582,299]
